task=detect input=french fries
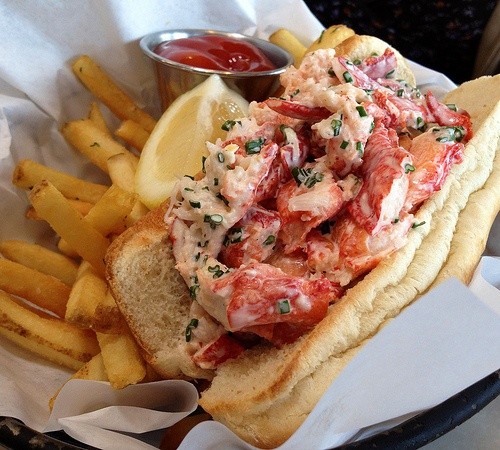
[0,25,355,410]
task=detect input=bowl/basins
[139,28,294,114]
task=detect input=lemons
[132,73,249,211]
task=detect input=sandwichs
[105,31,500,450]
